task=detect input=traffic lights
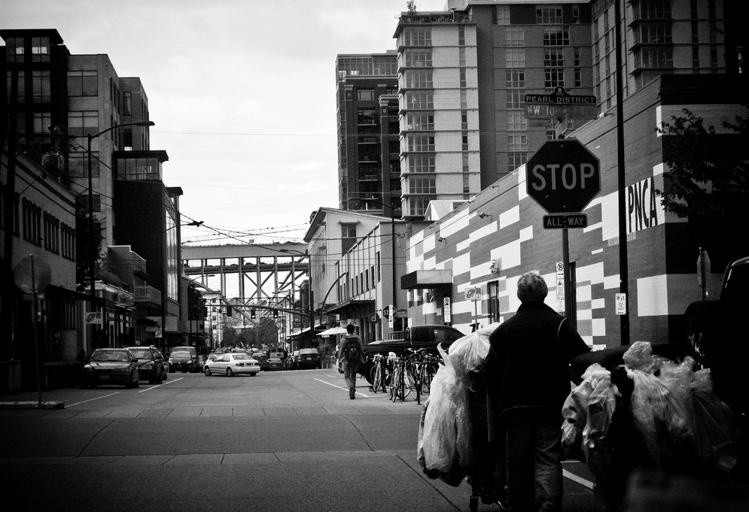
[250,309,256,320]
[273,309,278,318]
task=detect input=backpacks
[343,337,360,362]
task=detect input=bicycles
[366,344,443,407]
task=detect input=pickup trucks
[347,323,473,386]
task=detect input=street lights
[279,249,314,348]
[84,118,156,351]
[345,192,403,334]
[279,280,304,334]
[186,273,216,346]
[156,217,205,365]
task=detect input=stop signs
[524,137,602,215]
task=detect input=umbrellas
[315,326,349,346]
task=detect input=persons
[465,272,595,512]
[337,323,366,400]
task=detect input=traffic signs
[523,87,598,105]
[541,211,588,229]
[520,102,598,121]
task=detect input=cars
[74,338,324,390]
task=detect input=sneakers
[348,386,356,400]
